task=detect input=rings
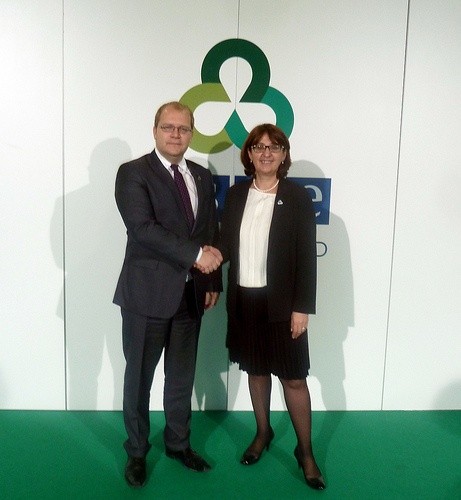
[302,328,305,329]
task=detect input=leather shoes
[166,447,211,472]
[125,455,146,488]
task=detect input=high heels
[296,447,325,490]
[240,431,274,465]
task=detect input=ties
[171,164,194,232]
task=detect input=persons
[193,123,326,490]
[111,102,225,488]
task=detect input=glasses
[250,143,284,153]
[157,123,190,134]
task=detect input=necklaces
[253,178,279,193]
